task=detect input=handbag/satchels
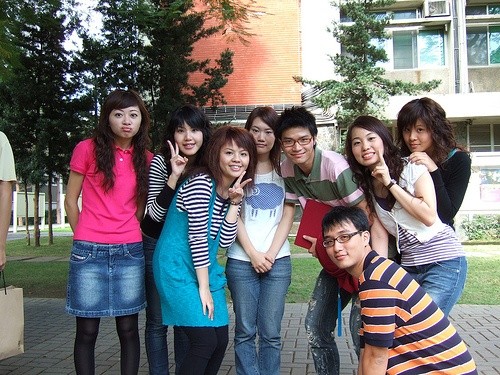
[0,271,24,360]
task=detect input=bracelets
[230,201,242,206]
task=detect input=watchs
[387,179,396,189]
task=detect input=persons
[64,89,154,375]
[277,106,375,375]
[152,125,258,375]
[388,97,472,265]
[225,106,296,374]
[0,131,17,270]
[140,106,212,375]
[321,205,478,375]
[346,115,467,318]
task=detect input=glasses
[322,231,360,247]
[281,136,313,147]
